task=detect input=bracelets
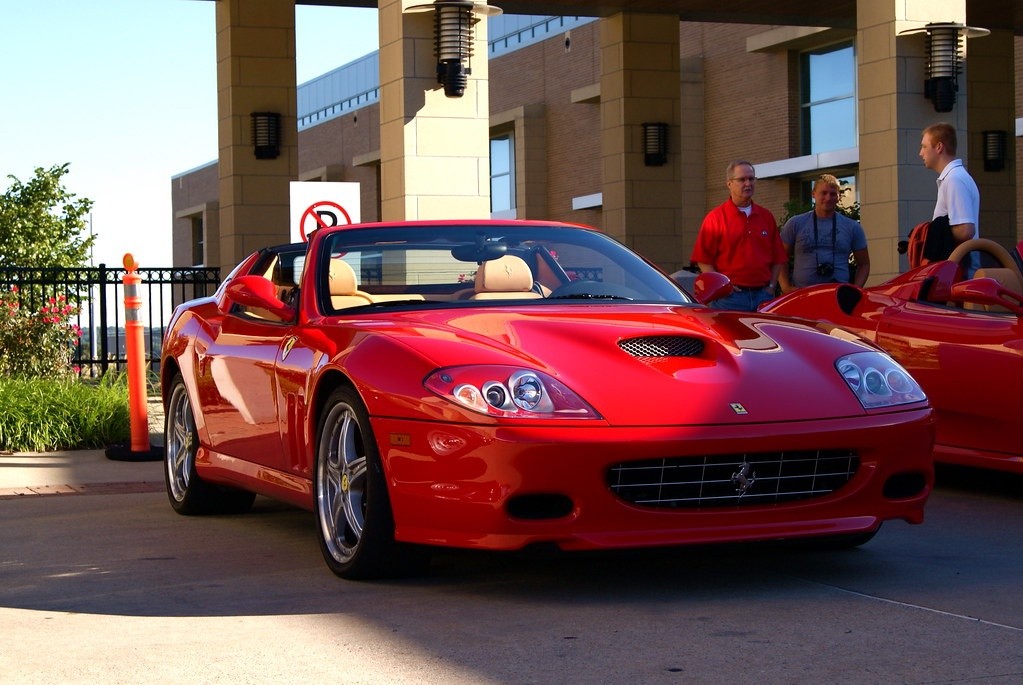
[769,283,775,288]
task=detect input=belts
[735,284,771,293]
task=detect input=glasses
[729,176,757,182]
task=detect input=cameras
[818,261,834,278]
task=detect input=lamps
[641,122,668,166]
[249,112,281,159]
[981,130,1007,171]
[898,21,991,114]
[405,0,504,99]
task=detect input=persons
[778,174,871,288]
[689,160,780,313]
[919,122,982,281]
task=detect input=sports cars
[156,216,935,581]
[757,235,1023,477]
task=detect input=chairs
[963,268,1023,313]
[468,254,544,300]
[298,258,376,310]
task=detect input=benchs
[373,286,475,303]
